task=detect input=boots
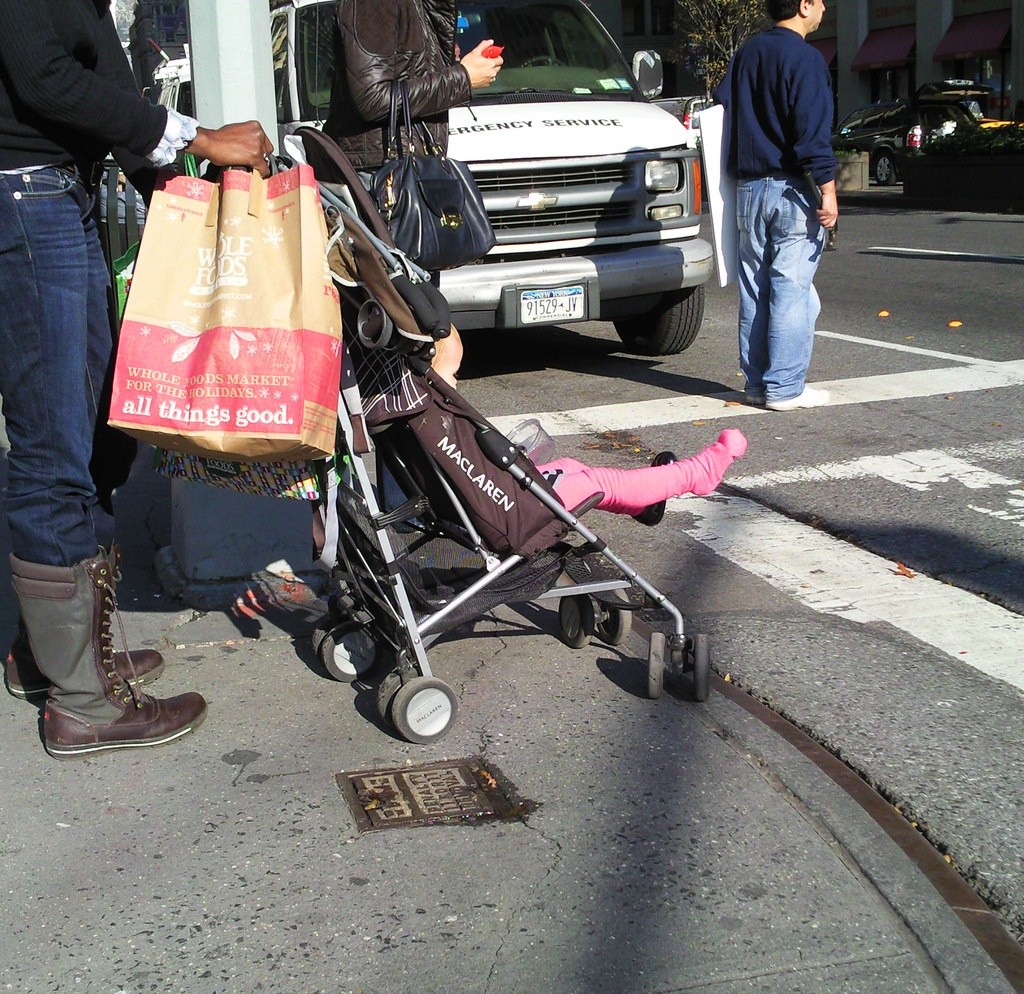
[4,540,209,763]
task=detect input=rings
[492,77,496,82]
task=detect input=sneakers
[765,385,832,411]
[745,386,765,404]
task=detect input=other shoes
[631,450,678,526]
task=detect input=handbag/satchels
[370,150,499,273]
[106,156,342,463]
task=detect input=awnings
[807,36,837,65]
[933,8,1012,64]
[851,23,916,72]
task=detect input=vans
[140,0,713,359]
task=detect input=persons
[0,0,276,759]
[430,323,747,528]
[325,0,503,537]
[709,0,842,411]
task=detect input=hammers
[804,169,838,252]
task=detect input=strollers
[260,124,715,746]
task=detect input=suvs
[831,77,994,187]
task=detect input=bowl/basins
[507,419,556,464]
